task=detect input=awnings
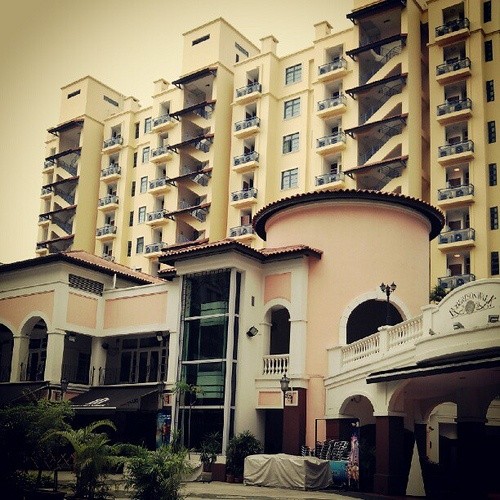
[65,384,164,414]
[0,380,50,388]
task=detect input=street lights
[380,281,397,325]
[279,374,290,452]
[60,377,69,400]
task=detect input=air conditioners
[315,55,348,190]
[144,114,169,255]
[231,83,262,241]
[33,157,58,250]
[95,136,120,259]
[435,19,475,297]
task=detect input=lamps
[488,314,499,324]
[155,332,163,342]
[453,322,464,331]
[69,333,77,343]
[248,326,258,337]
[280,374,290,410]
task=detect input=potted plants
[199,430,264,484]
